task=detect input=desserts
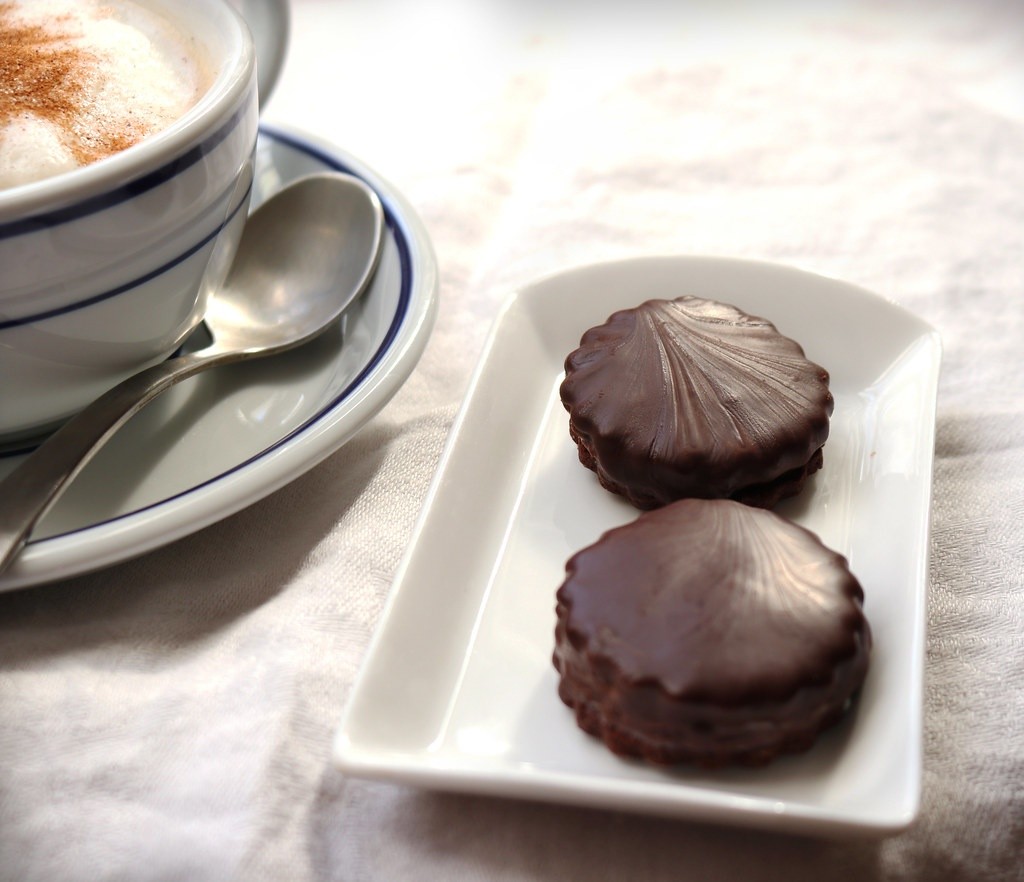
[559,290,835,508]
[552,496,872,768]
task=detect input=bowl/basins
[0,1,291,435]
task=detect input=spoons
[0,171,388,577]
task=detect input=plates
[333,256,944,844]
[0,120,443,592]
